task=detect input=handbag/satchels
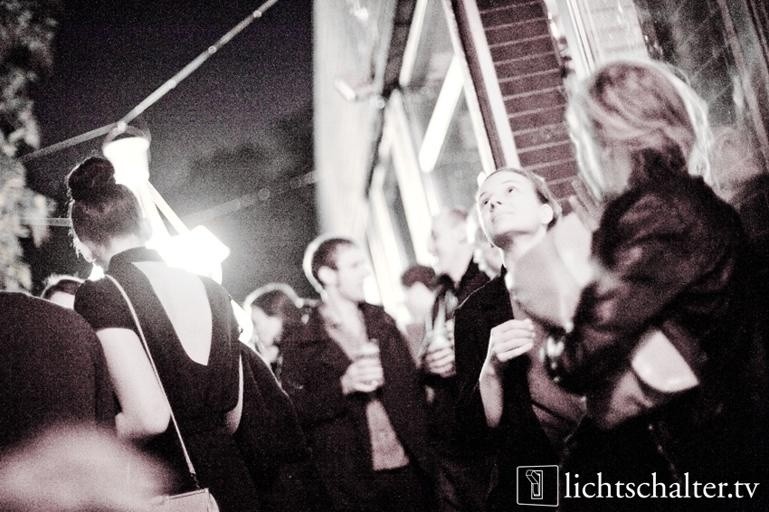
[152,488,220,512]
[505,211,699,427]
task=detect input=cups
[355,352,386,387]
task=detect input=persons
[454,166,606,505]
[68,156,260,512]
[41,205,503,512]
[538,61,769,512]
[0,293,121,452]
